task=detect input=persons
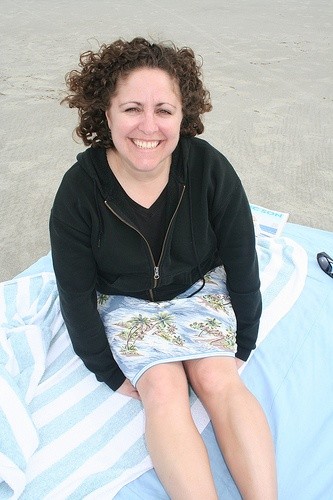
[48,36,277,499]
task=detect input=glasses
[316,252,333,278]
